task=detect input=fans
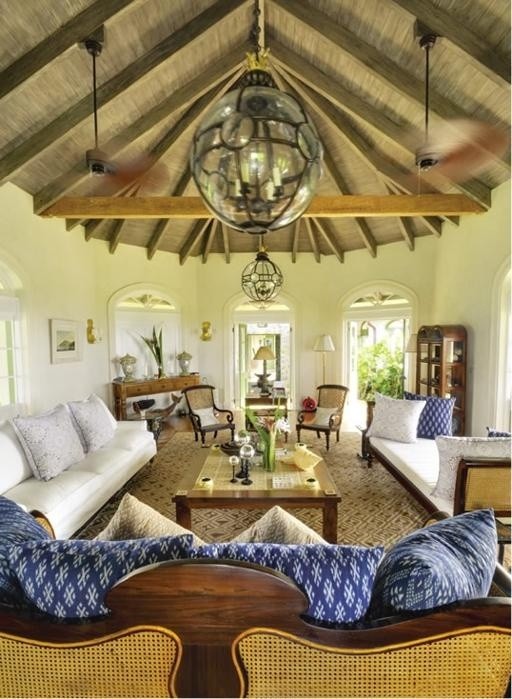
[39,24,170,202]
[363,18,506,198]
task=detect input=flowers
[246,401,288,438]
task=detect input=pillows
[309,406,338,426]
[429,427,511,502]
[366,390,455,443]
[11,397,115,481]
[192,406,219,427]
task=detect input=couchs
[0,502,511,698]
[362,391,511,564]
[0,395,157,542]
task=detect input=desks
[112,376,200,421]
[172,441,341,543]
[245,387,287,442]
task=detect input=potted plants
[140,326,167,380]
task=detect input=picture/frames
[50,319,79,364]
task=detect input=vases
[264,437,276,471]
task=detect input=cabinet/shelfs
[416,325,467,435]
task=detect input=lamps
[252,347,276,395]
[241,233,283,301]
[314,335,335,386]
[189,0,324,233]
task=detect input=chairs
[182,385,235,447]
[295,385,348,450]
